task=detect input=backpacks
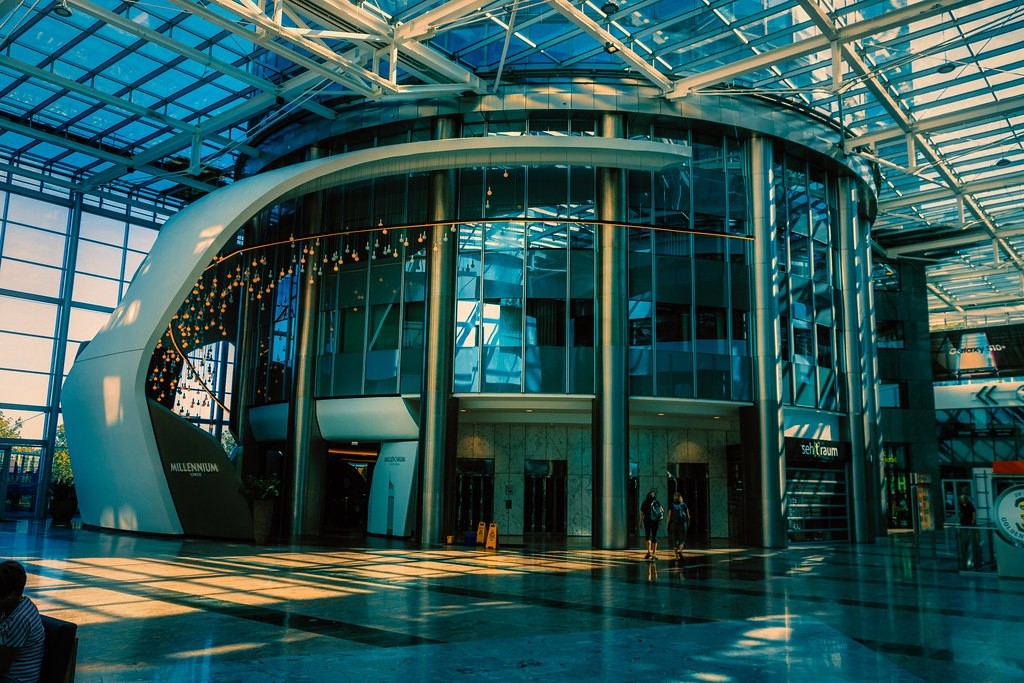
[672,503,686,525]
[649,500,664,522]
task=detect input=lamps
[936,59,956,75]
[53,0,73,17]
[994,152,1011,167]
[600,0,619,15]
[603,41,621,55]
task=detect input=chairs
[38,614,79,683]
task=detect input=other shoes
[645,552,658,561]
[675,551,685,560]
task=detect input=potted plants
[247,478,282,546]
[47,477,77,527]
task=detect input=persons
[666,492,690,560]
[959,495,976,526]
[640,491,664,558]
[0,559,46,683]
